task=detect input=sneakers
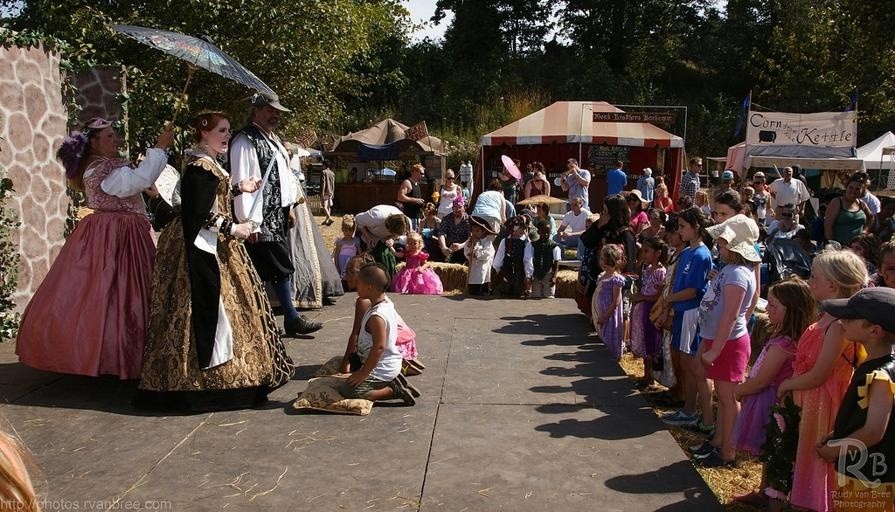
[401,358,426,377]
[322,219,334,226]
[389,372,422,406]
[632,375,738,469]
[731,489,784,512]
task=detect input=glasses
[781,212,794,217]
[535,179,543,182]
[753,181,762,185]
[627,196,638,202]
[418,170,424,175]
[447,177,455,180]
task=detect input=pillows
[291,376,377,416]
[311,356,353,377]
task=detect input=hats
[722,170,734,181]
[643,168,653,176]
[468,214,498,236]
[232,91,293,113]
[704,214,763,267]
[503,214,533,229]
[623,189,653,210]
[821,286,895,331]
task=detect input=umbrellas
[108,23,276,129]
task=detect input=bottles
[445,245,452,263]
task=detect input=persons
[319,159,336,226]
[15,116,175,383]
[334,154,895,512]
[131,109,297,409]
[227,97,325,337]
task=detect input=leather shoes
[284,313,323,335]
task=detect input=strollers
[762,233,811,284]
